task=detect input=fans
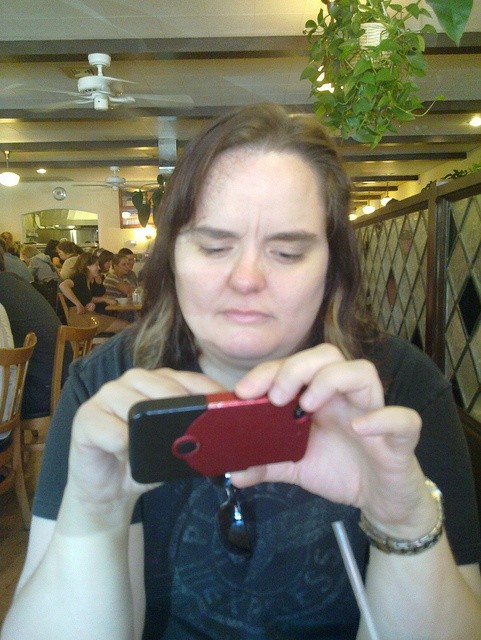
[5,53,196,121]
[70,166,163,200]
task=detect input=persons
[103,253,137,298]
[118,248,138,284]
[0,238,34,283]
[22,246,58,310]
[0,246,74,420]
[0,232,20,258]
[44,240,63,268]
[0,302,18,455]
[0,103,480,639]
[58,252,132,336]
[56,240,81,281]
[92,248,117,314]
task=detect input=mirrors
[21,207,101,252]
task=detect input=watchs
[358,476,445,556]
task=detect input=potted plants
[297,0,476,153]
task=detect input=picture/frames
[117,181,164,229]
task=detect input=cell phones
[128,392,312,483]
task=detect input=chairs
[0,330,39,532]
[20,312,101,489]
[55,290,116,362]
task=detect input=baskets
[342,21,395,70]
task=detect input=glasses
[213,472,257,555]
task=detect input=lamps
[0,150,21,188]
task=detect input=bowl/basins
[117,298,129,305]
